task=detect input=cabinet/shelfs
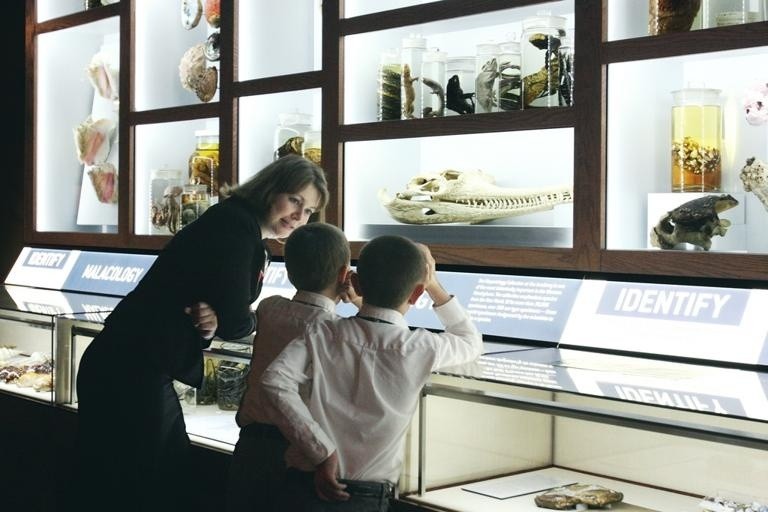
[24,2,768,289]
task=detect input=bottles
[374,15,574,121]
[180,184,210,231]
[189,130,219,196]
[669,87,725,194]
[149,169,183,236]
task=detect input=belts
[337,479,402,502]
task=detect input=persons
[219,221,363,510]
[255,231,487,512]
[71,152,329,511]
